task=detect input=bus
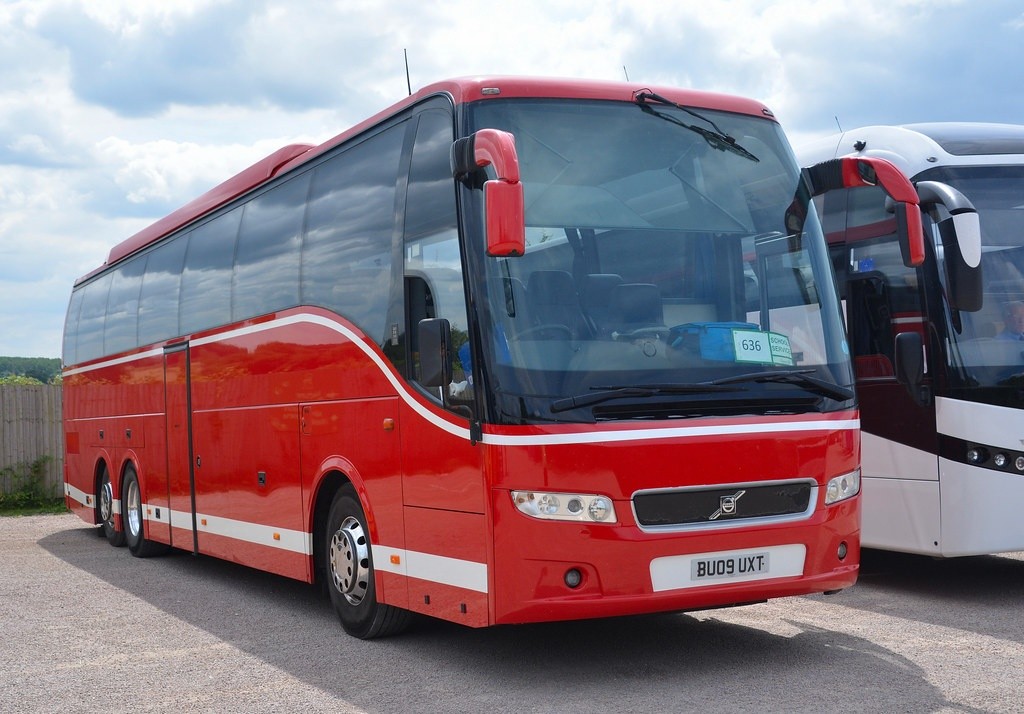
[57,74,1023,638]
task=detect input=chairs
[331,266,663,338]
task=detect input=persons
[458,279,533,398]
[994,300,1024,341]
